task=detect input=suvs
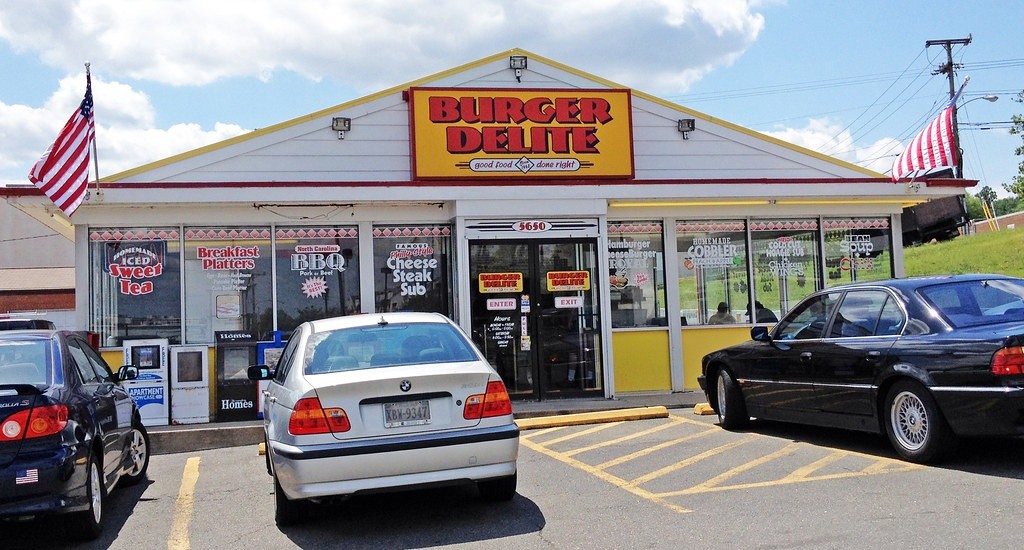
[0,314,58,331]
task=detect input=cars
[247,310,520,527]
[1,328,150,544]
[698,275,1024,465]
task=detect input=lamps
[510,56,528,82]
[677,118,695,140]
[331,117,351,140]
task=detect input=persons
[745,301,778,323]
[708,302,736,325]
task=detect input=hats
[745,300,760,316]
[718,302,730,308]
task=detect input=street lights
[951,94,999,234]
[380,267,393,312]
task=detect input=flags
[28,75,95,218]
[893,85,964,183]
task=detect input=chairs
[370,353,403,367]
[401,337,446,360]
[0,363,40,383]
[314,341,360,371]
